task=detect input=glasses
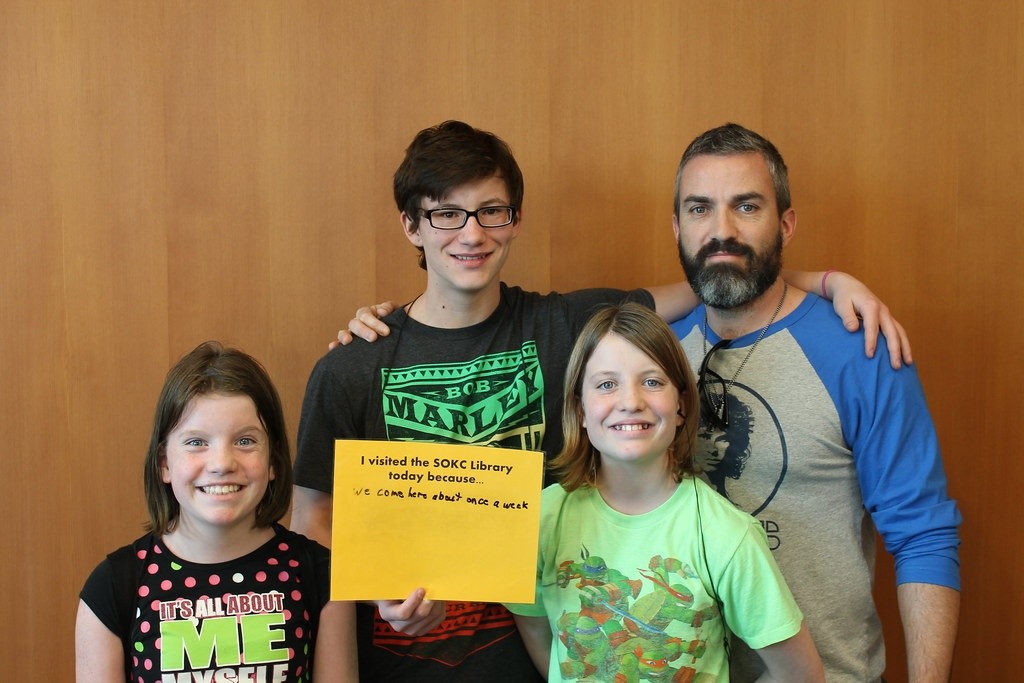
[695,337,732,430]
[415,206,515,230]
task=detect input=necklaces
[702,275,788,432]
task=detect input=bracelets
[822,269,844,300]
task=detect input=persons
[289,120,913,683]
[326,122,962,683]
[500,299,825,682]
[74,344,359,683]
